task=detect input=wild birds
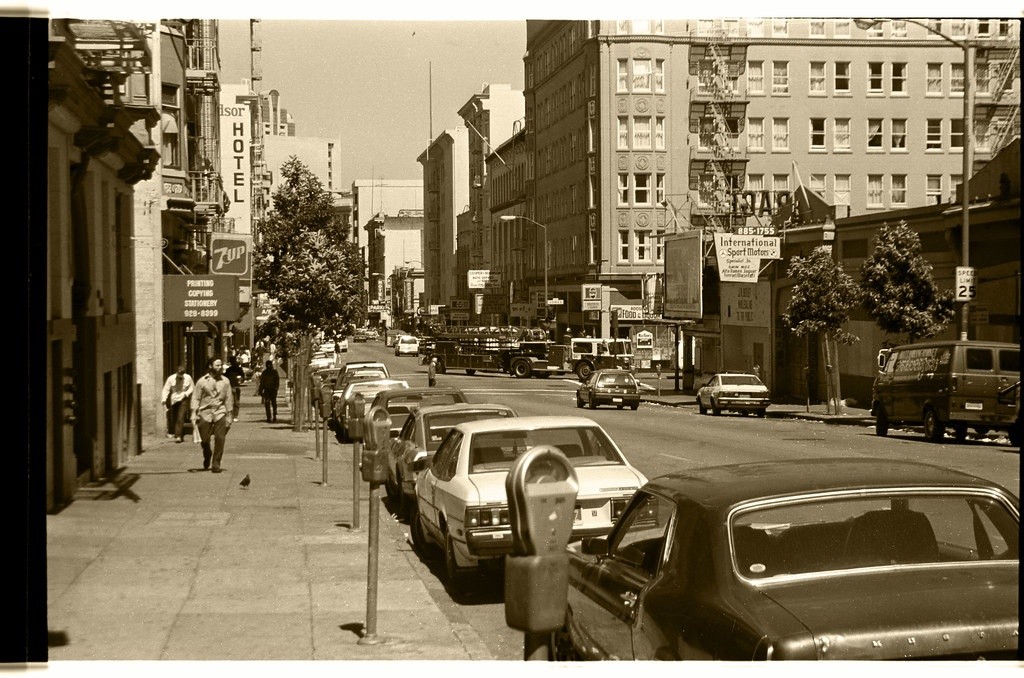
[239,473,251,490]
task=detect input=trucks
[418,326,633,379]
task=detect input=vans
[872,340,1020,446]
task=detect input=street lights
[500,215,548,322]
[406,260,432,316]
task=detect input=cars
[410,415,659,591]
[576,369,641,410]
[555,458,1019,661]
[308,328,528,518]
[394,335,420,357]
[696,373,771,417]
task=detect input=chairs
[472,446,504,466]
[642,509,939,590]
[554,444,583,457]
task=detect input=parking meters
[753,365,761,378]
[347,392,366,533]
[319,384,333,487]
[656,364,662,398]
[504,444,580,661]
[357,407,391,646]
[311,375,323,461]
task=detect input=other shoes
[203,452,212,468]
[176,437,181,443]
[212,467,222,474]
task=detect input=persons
[258,361,280,423]
[428,358,438,387]
[189,357,233,473]
[333,341,342,365]
[228,338,265,383]
[161,366,194,442]
[224,356,244,423]
